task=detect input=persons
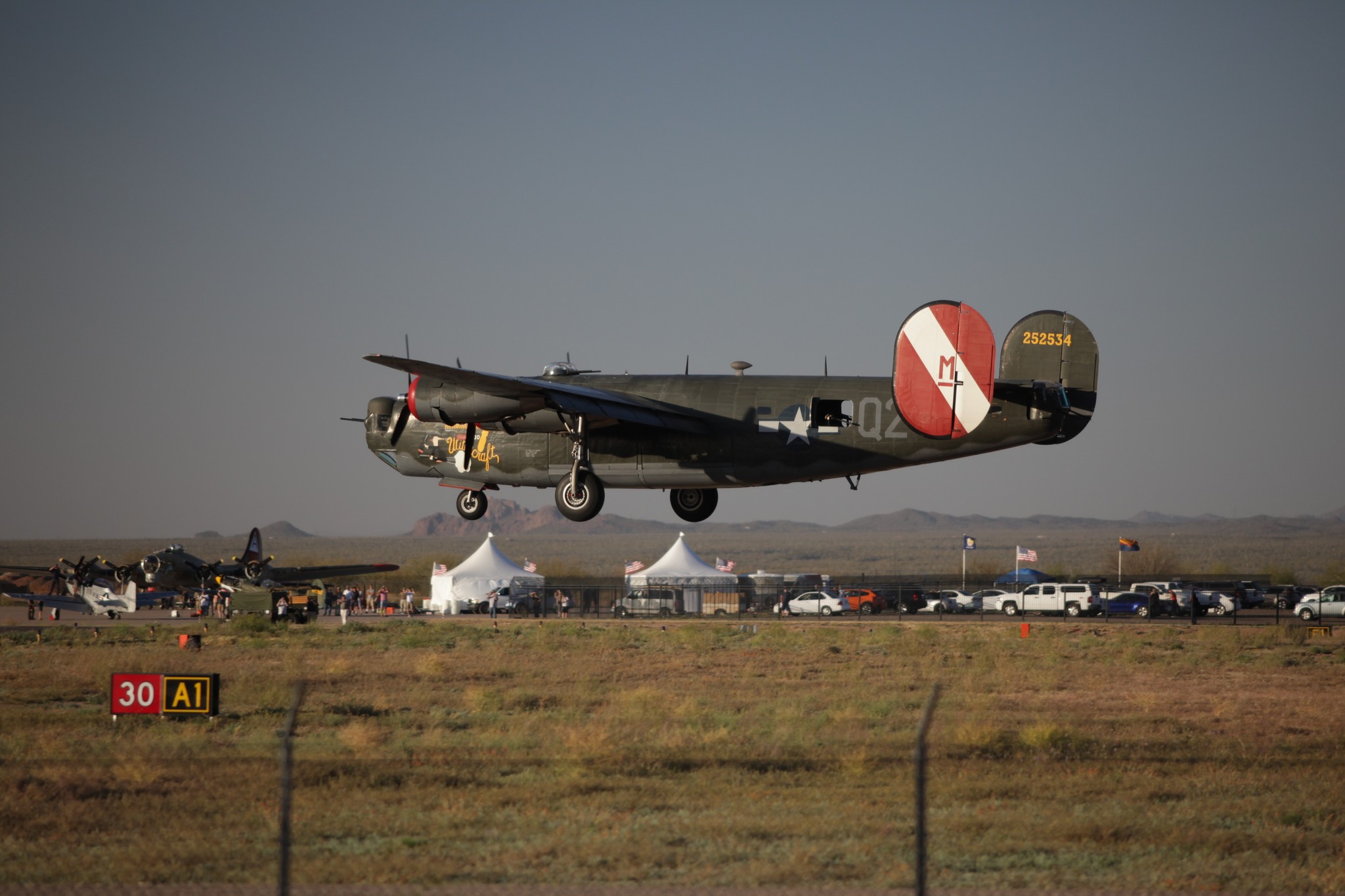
[26,563,417,625]
[487,588,572,618]
[1147,588,1200,623]
[776,584,845,617]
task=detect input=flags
[432,561,447,575]
[1118,537,1140,552]
[624,558,646,574]
[715,556,737,573]
[964,534,978,550]
[524,558,537,573]
[1017,546,1037,563]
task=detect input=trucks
[226,590,308,626]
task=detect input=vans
[995,582,1100,617]
[609,587,684,620]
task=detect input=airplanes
[1,527,401,623]
[332,297,1100,522]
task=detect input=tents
[624,532,738,596]
[432,532,546,615]
[994,567,1055,591]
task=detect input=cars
[916,591,953,615]
[504,585,574,614]
[968,588,1009,614]
[1096,580,1345,622]
[468,585,509,615]
[867,588,928,615]
[929,589,982,614]
[834,588,887,615]
[772,589,850,616]
